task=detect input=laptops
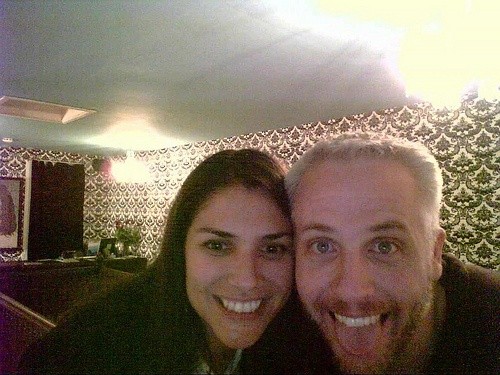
[83,238,117,258]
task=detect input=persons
[17,149,295,375]
[241,132,500,375]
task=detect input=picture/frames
[0,176,26,254]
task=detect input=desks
[0,255,148,322]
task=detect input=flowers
[112,220,142,256]
